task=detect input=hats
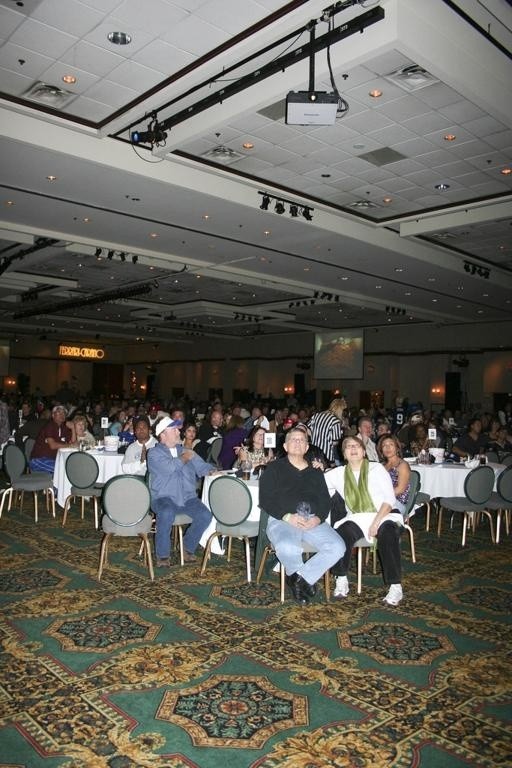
[155,417,181,437]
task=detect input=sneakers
[382,584,404,607]
[176,542,197,563]
[157,558,170,568]
[334,575,349,599]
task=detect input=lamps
[95,248,140,264]
[178,321,207,338]
[258,191,315,222]
[288,290,340,308]
[464,261,493,279]
[233,311,266,323]
[385,305,409,317]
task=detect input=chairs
[0,432,58,520]
[256,469,430,604]
[96,474,158,582]
[476,465,512,543]
[437,466,495,547]
[146,472,194,567]
[484,451,512,463]
[199,477,260,583]
[193,437,227,467]
[62,452,105,531]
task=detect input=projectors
[284,92,339,127]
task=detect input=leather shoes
[286,574,317,605]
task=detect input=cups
[296,502,310,521]
[241,460,252,480]
[480,454,485,466]
[418,450,429,464]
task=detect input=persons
[312,436,403,605]
[376,433,411,515]
[146,416,224,567]
[259,428,347,606]
[1,394,512,502]
